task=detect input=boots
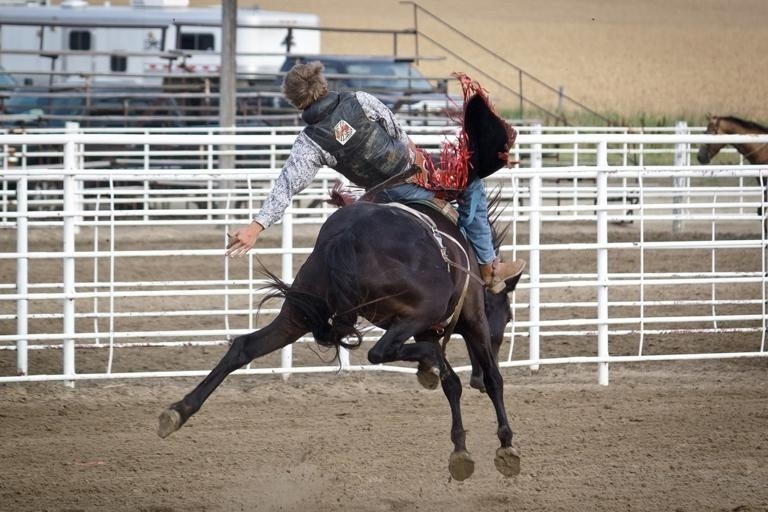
[481,259,526,294]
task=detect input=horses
[696,112,768,238]
[156,180,524,482]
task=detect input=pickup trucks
[164,56,461,127]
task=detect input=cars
[1,64,187,131]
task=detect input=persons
[224,60,527,296]
